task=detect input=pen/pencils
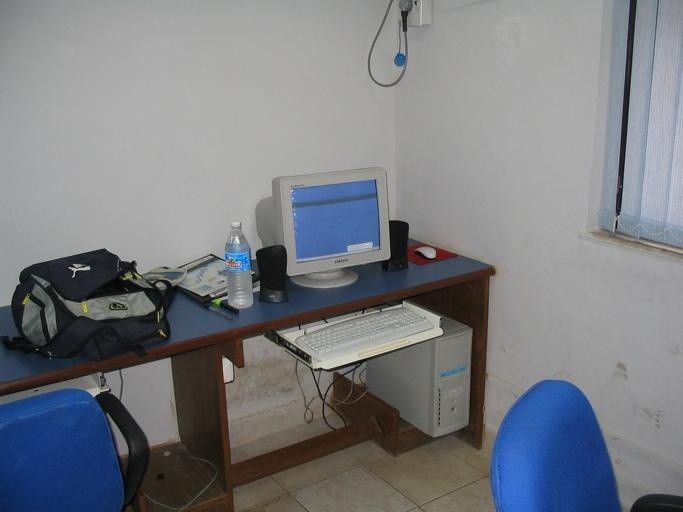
[202,303,233,320]
[213,298,239,314]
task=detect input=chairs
[0,387,151,512]
[489,378,682,512]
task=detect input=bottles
[224,223,253,308]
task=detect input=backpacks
[2,247,175,360]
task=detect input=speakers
[385,220,410,271]
[256,245,288,303]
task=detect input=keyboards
[296,307,434,362]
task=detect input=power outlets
[399,0,433,28]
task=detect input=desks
[0,239,498,510]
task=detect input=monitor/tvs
[271,168,391,289]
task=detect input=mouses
[415,247,436,259]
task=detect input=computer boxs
[365,299,473,438]
